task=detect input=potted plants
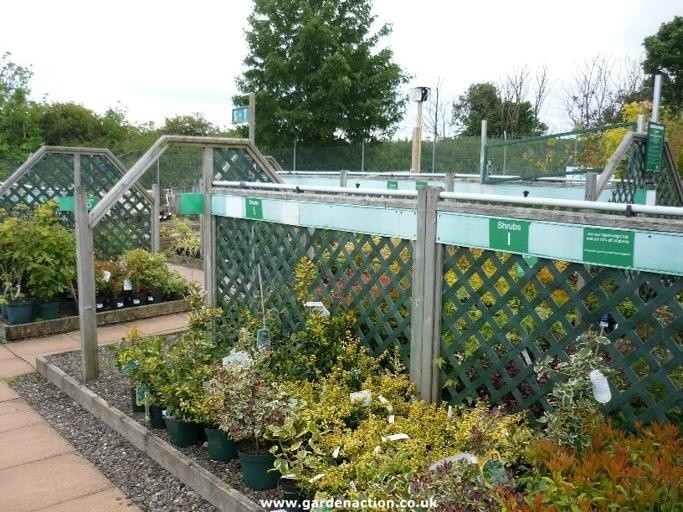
[0,202,201,323]
